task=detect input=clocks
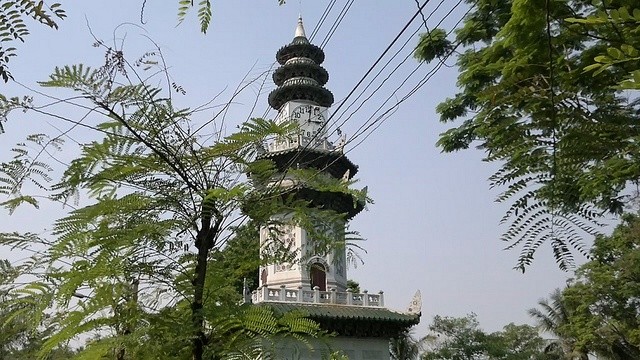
[293,104,326,138]
